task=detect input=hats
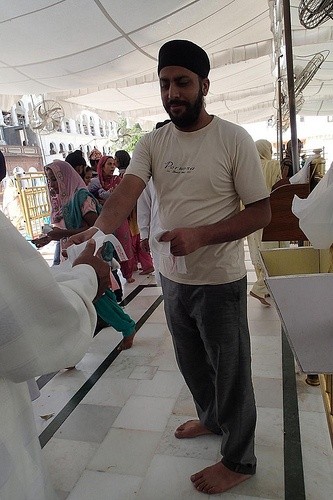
[158,40,210,80]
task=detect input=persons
[32,148,154,369]
[0,151,110,500]
[62,39,272,494]
[238,139,306,307]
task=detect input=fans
[268,0,333,134]
[2,100,65,146]
[108,128,132,150]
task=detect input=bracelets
[90,226,100,231]
